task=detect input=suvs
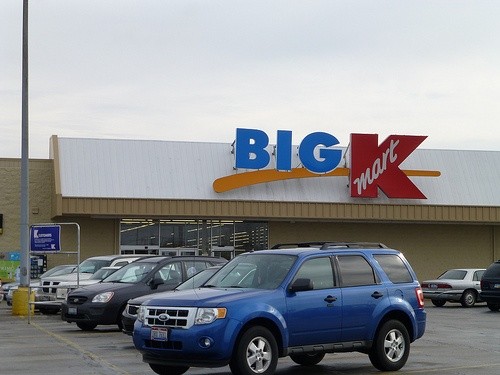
[132,240,426,375]
[479,259,500,312]
[40,254,157,304]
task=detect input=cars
[62,255,229,329]
[2,264,78,306]
[56,266,175,304]
[420,266,488,308]
[121,263,257,335]
